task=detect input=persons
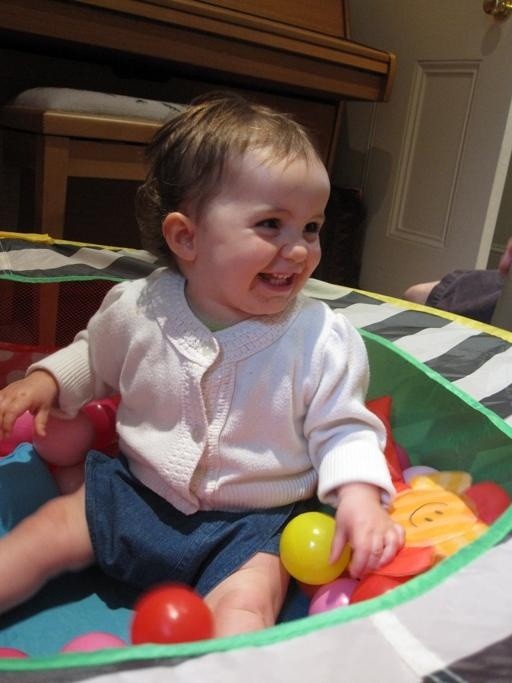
[403,238,512,333]
[0,91,404,641]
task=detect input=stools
[6,84,190,343]
